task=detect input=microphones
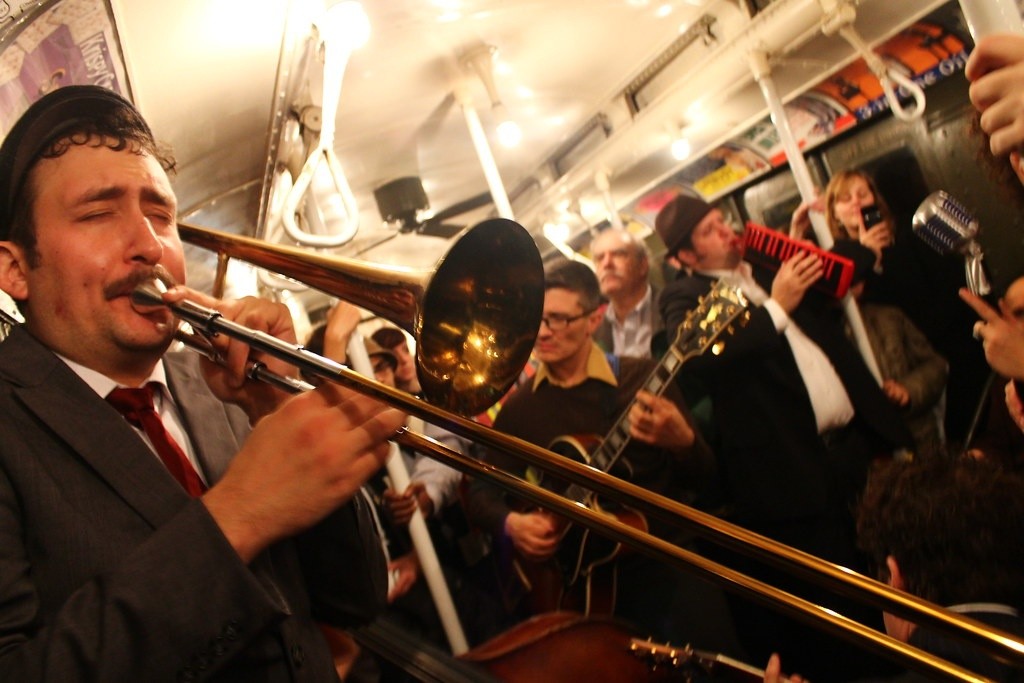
[913,190,1002,318]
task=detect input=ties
[104,383,208,501]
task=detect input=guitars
[490,277,752,619]
[628,603,1024,683]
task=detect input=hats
[0,83,157,239]
[545,259,611,303]
[655,194,719,260]
[298,321,398,385]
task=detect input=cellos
[344,611,659,683]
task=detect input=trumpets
[177,217,546,418]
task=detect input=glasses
[543,312,586,331]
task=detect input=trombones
[130,274,1024,683]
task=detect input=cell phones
[861,205,883,231]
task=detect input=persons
[299,30,1024,683]
[0,83,408,683]
